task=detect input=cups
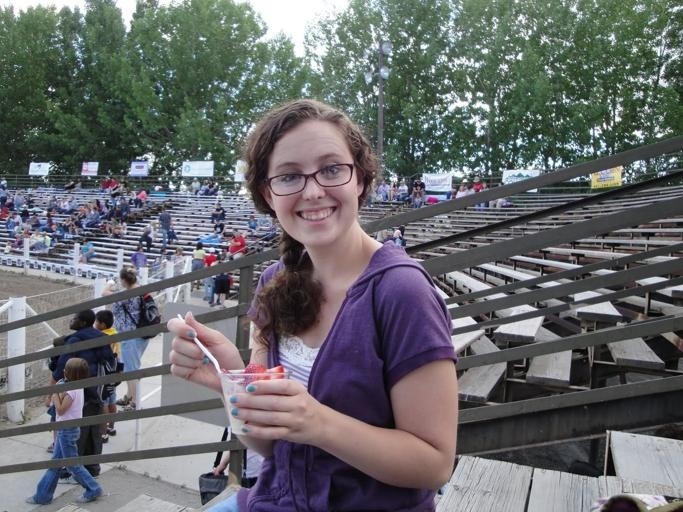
[215,370,291,436]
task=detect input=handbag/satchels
[47,335,70,370]
[199,474,257,506]
[96,357,124,401]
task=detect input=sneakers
[26,395,135,504]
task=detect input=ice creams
[219,363,283,436]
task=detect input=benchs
[0,179,683,511]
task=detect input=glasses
[265,164,354,196]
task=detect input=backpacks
[122,294,160,339]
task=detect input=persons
[447,179,507,208]
[95,310,122,444]
[172,203,277,306]
[190,178,218,196]
[47,309,112,477]
[377,224,405,246]
[134,209,179,267]
[365,177,425,208]
[168,101,458,512]
[103,267,149,412]
[0,177,149,263]
[26,357,104,504]
